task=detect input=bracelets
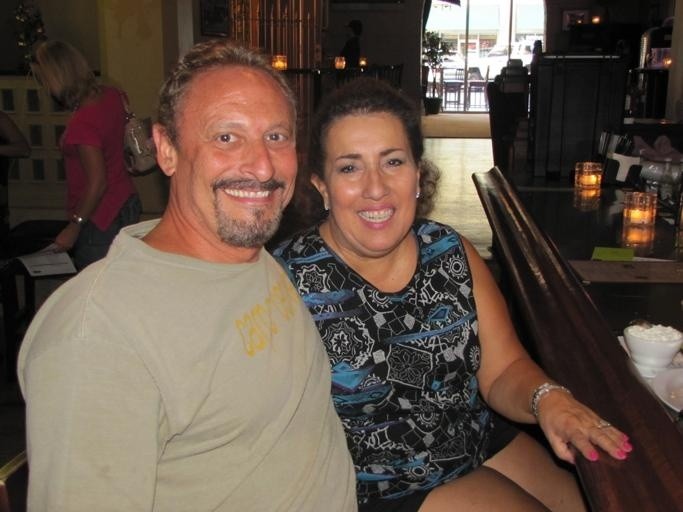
[72,213,87,226]
[532,382,572,419]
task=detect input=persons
[17,36,357,512]
[27,37,143,269]
[271,79,632,512]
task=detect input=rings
[597,421,611,429]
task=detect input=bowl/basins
[623,323,683,367]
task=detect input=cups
[622,223,655,253]
[574,162,602,188]
[333,55,348,70]
[269,54,288,72]
[574,190,600,210]
[358,54,368,68]
[621,191,657,222]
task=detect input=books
[1,242,77,275]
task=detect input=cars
[426,42,533,95]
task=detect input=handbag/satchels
[118,90,157,176]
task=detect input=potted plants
[420,31,454,114]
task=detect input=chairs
[427,66,494,106]
[1,218,109,329]
